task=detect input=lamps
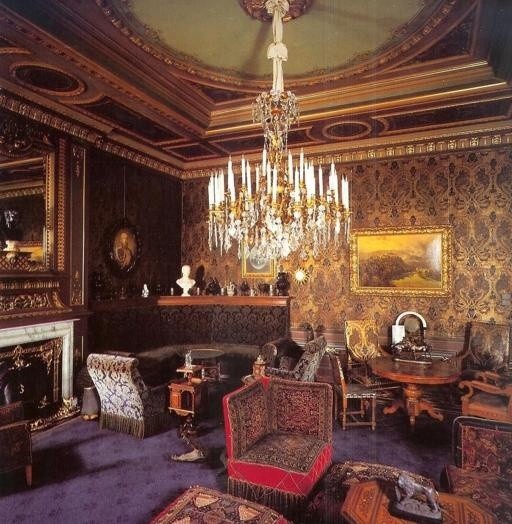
[206,0,354,264]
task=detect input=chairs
[459,369,512,423]
[222,375,339,499]
[88,352,170,439]
[250,334,329,381]
[452,319,511,379]
[343,319,397,413]
[452,414,512,516]
[329,347,378,430]
[0,401,33,486]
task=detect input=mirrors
[0,106,67,280]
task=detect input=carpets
[147,482,285,524]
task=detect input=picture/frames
[102,218,143,278]
[349,224,453,298]
[240,237,275,279]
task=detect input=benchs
[90,296,293,366]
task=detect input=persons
[115,233,133,267]
[175,265,197,288]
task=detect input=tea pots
[225,282,235,297]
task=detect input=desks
[171,348,226,432]
[368,353,460,426]
[341,479,498,524]
